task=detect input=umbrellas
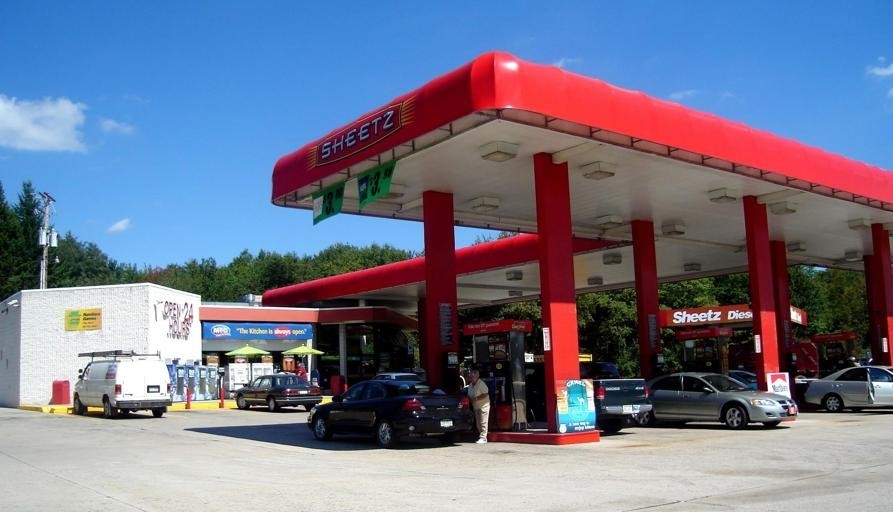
[280,344,325,363]
[225,344,271,363]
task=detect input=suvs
[73,352,173,417]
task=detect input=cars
[648,366,892,430]
[235,374,322,412]
[308,380,473,448]
[372,372,418,382]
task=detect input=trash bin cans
[52,380,70,404]
[330,375,345,396]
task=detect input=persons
[296,361,307,380]
[468,370,491,444]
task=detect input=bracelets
[476,398,477,401]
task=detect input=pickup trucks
[524,360,652,436]
